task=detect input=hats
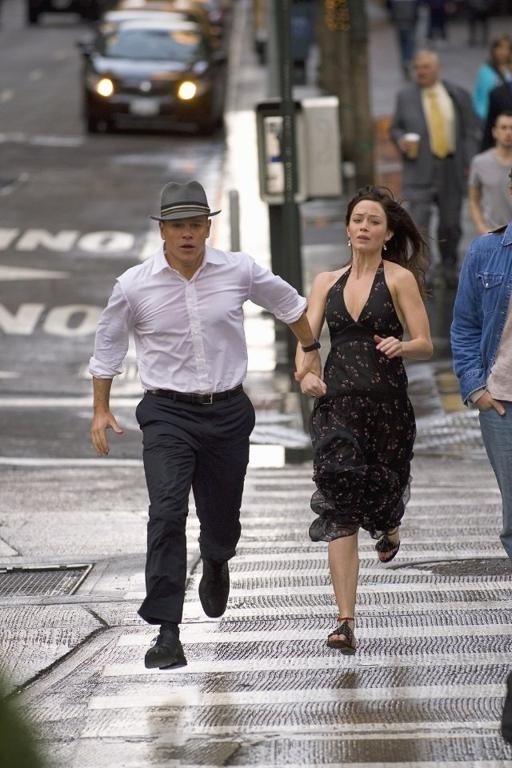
[151,181,222,221]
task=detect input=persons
[373,1,512,276]
[449,220,511,559]
[87,180,322,671]
[294,187,434,657]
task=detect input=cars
[28,0,227,140]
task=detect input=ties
[426,88,448,158]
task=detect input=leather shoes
[199,557,230,617]
[145,635,187,669]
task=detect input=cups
[403,133,421,160]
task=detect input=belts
[147,384,242,404]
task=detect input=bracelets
[301,339,321,353]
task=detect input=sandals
[326,617,357,655]
[374,527,400,563]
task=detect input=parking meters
[256,97,345,337]
